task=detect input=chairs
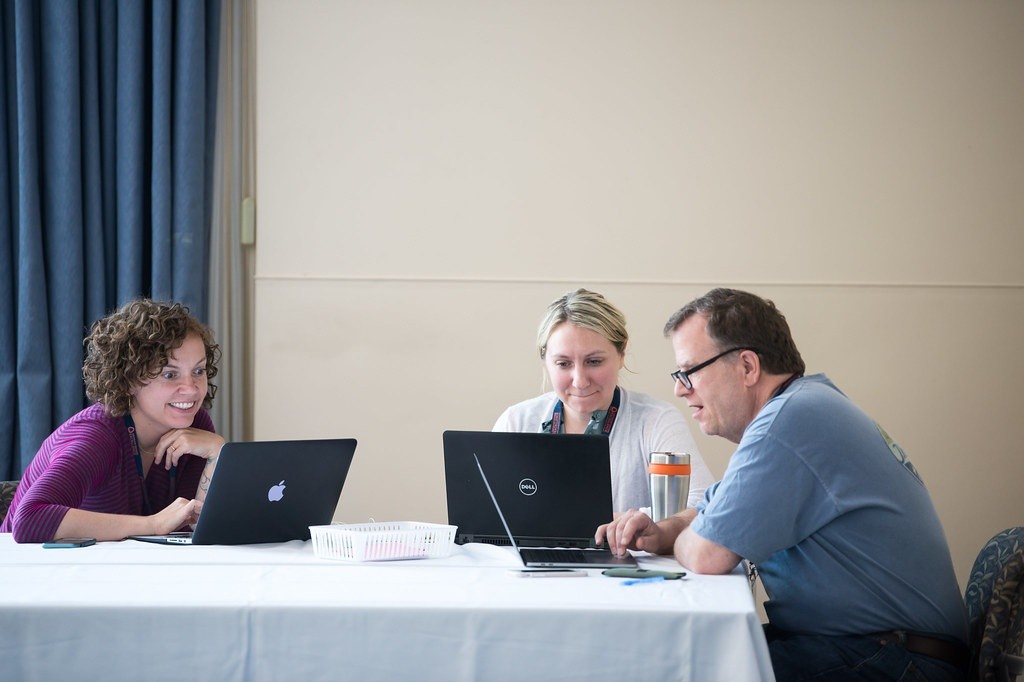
[964,527,1024,682]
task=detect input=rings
[169,444,176,451]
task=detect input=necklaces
[139,444,154,455]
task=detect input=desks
[0,533,776,682]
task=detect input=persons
[492,288,717,523]
[596,287,971,682]
[0,297,225,543]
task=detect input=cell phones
[43,537,96,548]
[602,567,686,580]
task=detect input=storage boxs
[308,521,459,561]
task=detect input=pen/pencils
[598,566,688,582]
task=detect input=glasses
[670,346,759,389]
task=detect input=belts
[878,631,976,674]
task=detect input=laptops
[473,453,638,568]
[127,438,358,544]
[443,430,614,549]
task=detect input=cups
[648,451,690,524]
